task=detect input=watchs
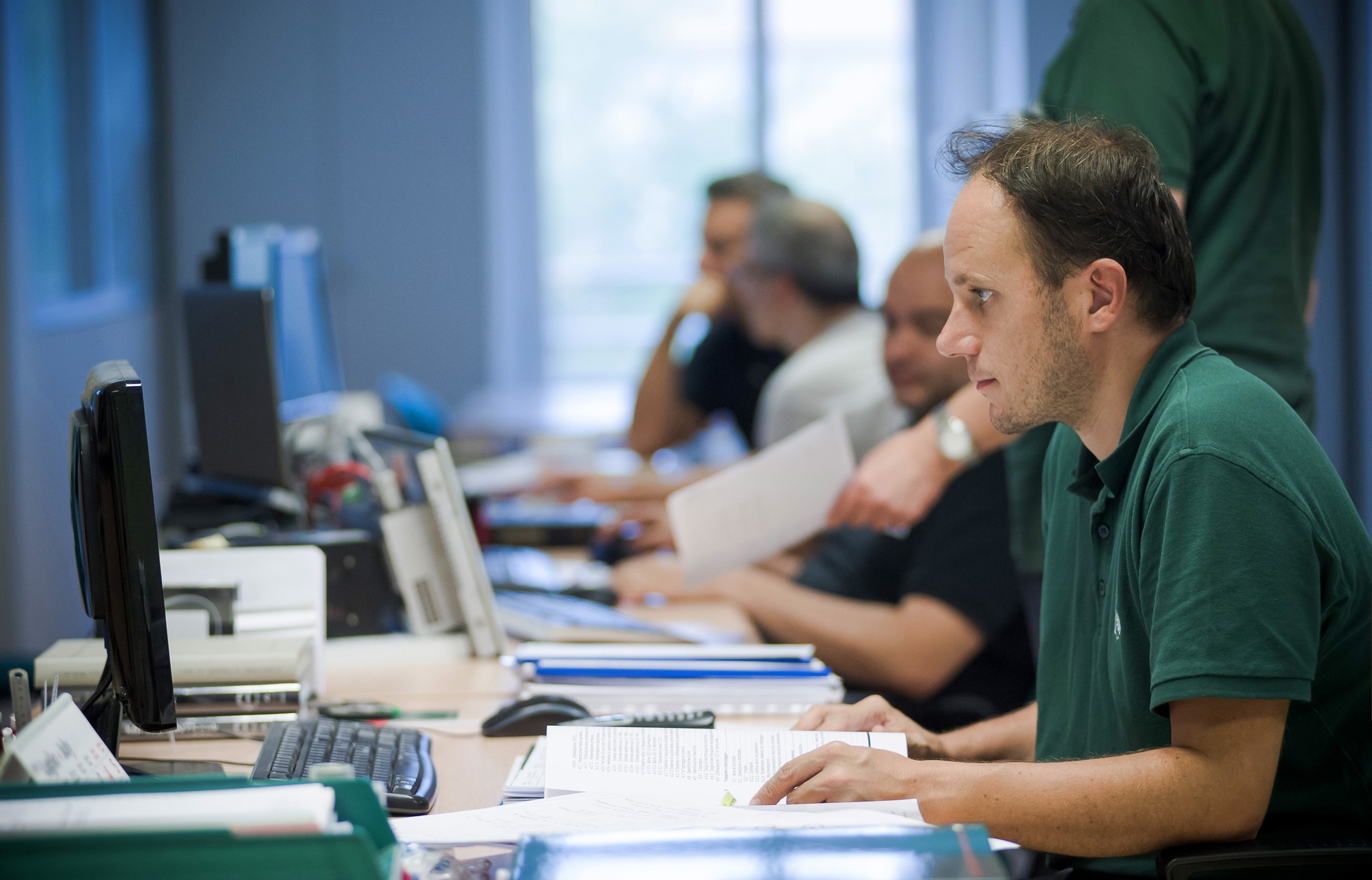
[930,402,982,471]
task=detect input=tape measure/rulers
[8,666,34,736]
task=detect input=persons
[511,187,910,513]
[746,112,1372,880]
[606,228,1047,735]
[620,171,825,455]
[822,0,1332,692]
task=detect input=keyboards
[253,718,438,815]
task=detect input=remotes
[559,711,716,729]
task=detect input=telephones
[555,709,718,729]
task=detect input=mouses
[481,698,591,737]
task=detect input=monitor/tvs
[180,224,342,493]
[66,356,182,734]
